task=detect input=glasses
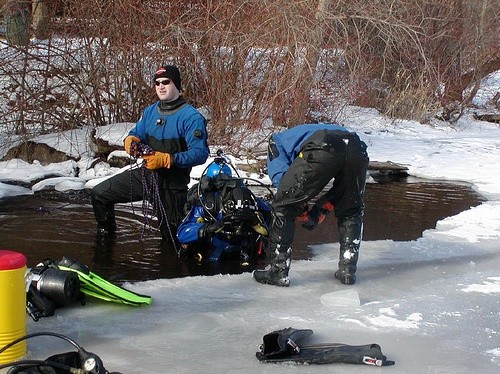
[155,79,172,86]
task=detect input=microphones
[217,149,230,164]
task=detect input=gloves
[198,223,224,238]
[124,136,140,154]
[141,152,171,169]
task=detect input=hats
[154,65,181,93]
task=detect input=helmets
[207,163,232,179]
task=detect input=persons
[254,124,369,287]
[92,65,209,280]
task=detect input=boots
[335,211,364,284]
[255,213,294,286]
